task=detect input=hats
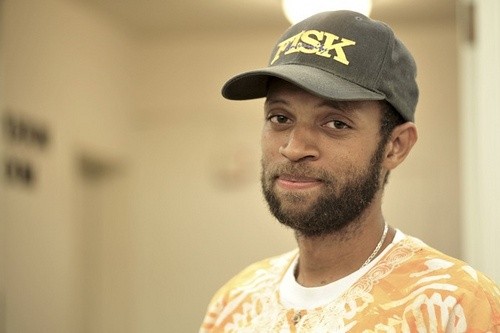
[222,10,419,123]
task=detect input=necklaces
[361,221,389,270]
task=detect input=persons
[198,10,500,333]
